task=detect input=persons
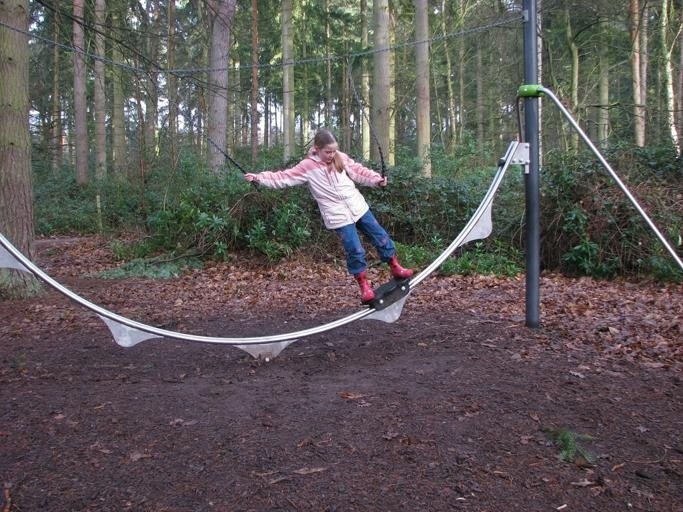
[243,127,413,305]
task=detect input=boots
[353,272,374,303]
[387,256,413,278]
